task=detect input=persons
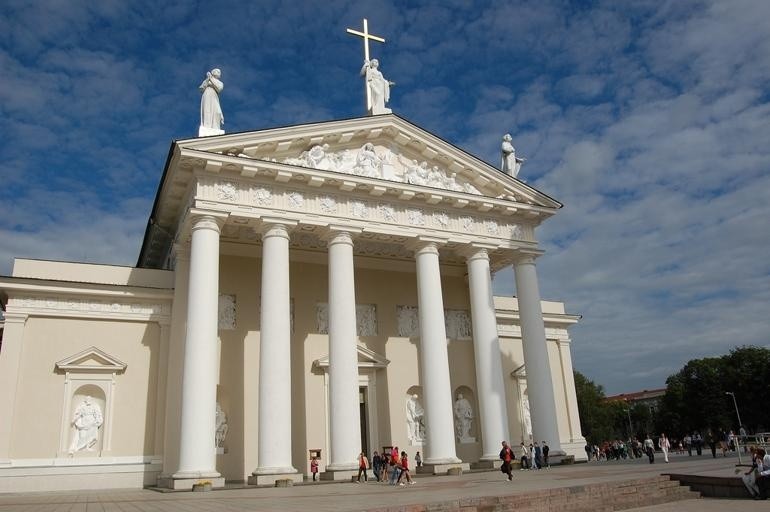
[406,393,423,440]
[453,392,474,439]
[355,444,422,486]
[534,442,543,471]
[501,133,526,178]
[584,425,770,464]
[359,57,397,108]
[199,67,225,128]
[519,442,530,472]
[254,141,485,199]
[216,403,229,446]
[70,394,104,455]
[755,447,770,501]
[310,457,319,482]
[530,444,537,470]
[744,444,762,499]
[542,441,551,470]
[500,441,515,482]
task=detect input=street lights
[621,396,635,443]
[725,390,747,434]
